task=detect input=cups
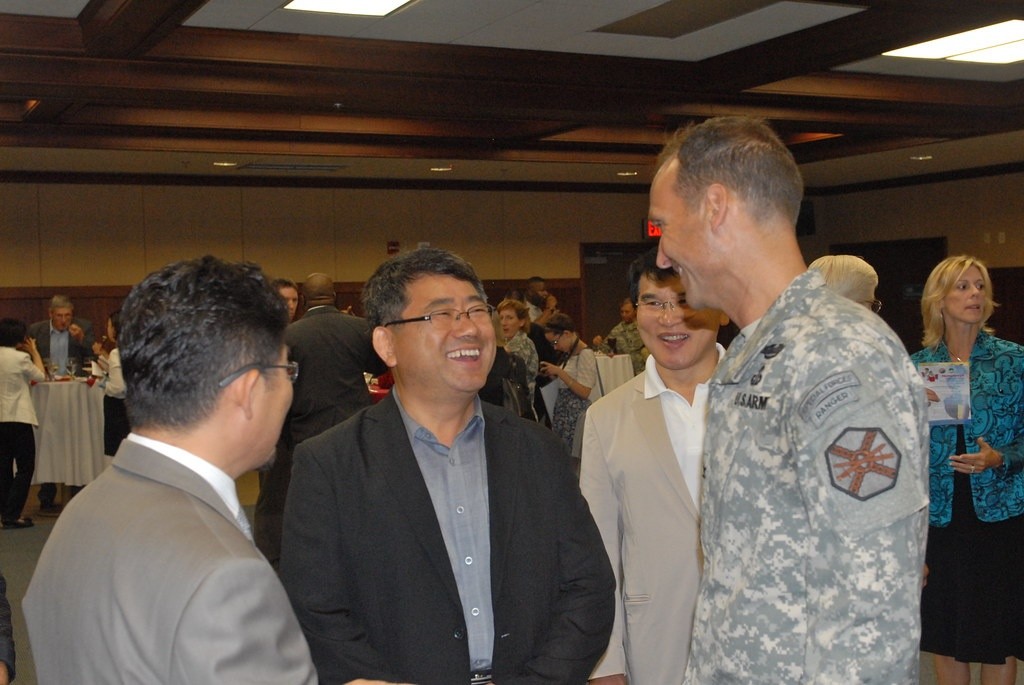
[608,338,616,354]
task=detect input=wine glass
[64,357,78,380]
[43,358,59,381]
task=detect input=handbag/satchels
[503,351,534,417]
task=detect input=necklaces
[940,340,962,362]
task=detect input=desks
[13,379,115,516]
[588,353,634,402]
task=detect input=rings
[971,465,975,471]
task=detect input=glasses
[385,303,495,327]
[866,299,881,313]
[549,333,563,346]
[635,297,696,318]
[217,360,300,388]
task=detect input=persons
[1,316,46,530]
[23,295,98,515]
[808,254,881,313]
[18,255,317,685]
[0,573,15,685]
[577,244,731,685]
[907,256,1024,685]
[645,114,929,685]
[92,310,133,458]
[279,244,618,685]
[255,279,300,560]
[284,272,650,461]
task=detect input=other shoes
[1,517,35,528]
